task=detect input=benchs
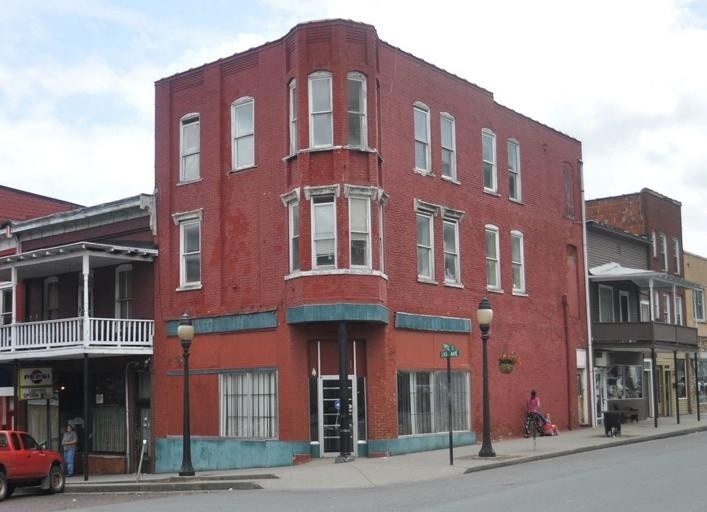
[618,407,640,423]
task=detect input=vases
[499,363,513,373]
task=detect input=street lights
[477,295,499,458]
[178,311,197,476]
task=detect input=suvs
[0,429,64,502]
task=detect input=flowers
[496,352,520,364]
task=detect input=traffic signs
[439,349,459,357]
[441,342,455,352]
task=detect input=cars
[677,375,707,397]
[606,376,630,396]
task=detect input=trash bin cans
[603,411,624,437]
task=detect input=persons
[61,423,79,478]
[544,412,550,424]
[523,389,542,437]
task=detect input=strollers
[535,410,560,436]
